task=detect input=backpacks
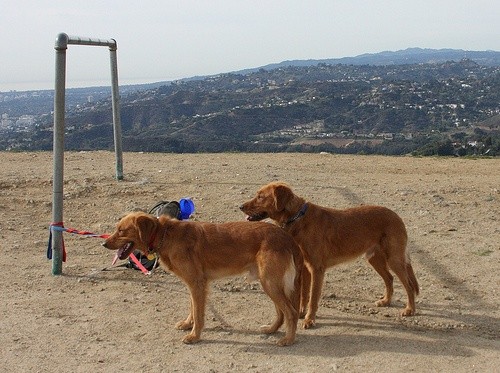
[126,200,180,271]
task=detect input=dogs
[238,180,420,329]
[101,211,304,347]
